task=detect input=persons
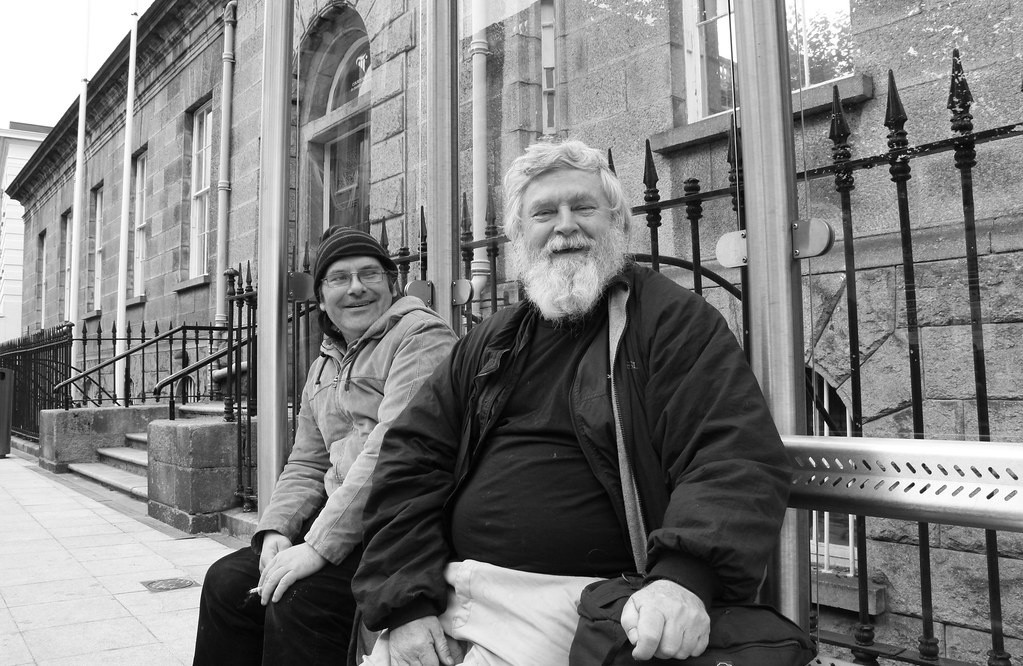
[349,139,793,666]
[192,223,460,666]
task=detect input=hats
[312,225,399,302]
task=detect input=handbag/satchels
[568,571,819,666]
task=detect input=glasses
[320,269,389,288]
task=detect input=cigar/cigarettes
[250,586,262,594]
